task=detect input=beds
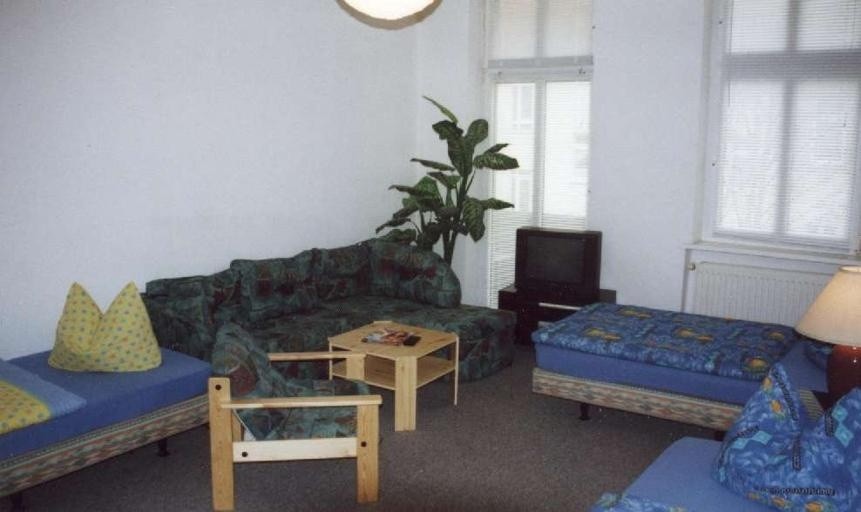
[0,347,207,511]
[533,300,800,442]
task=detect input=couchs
[141,239,515,383]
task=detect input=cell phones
[404,335,420,345]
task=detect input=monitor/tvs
[515,226,602,302]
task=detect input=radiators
[689,261,832,331]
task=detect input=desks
[325,319,461,432]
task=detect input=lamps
[795,265,861,403]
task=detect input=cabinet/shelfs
[499,284,617,346]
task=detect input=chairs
[208,322,382,511]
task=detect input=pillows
[716,362,858,512]
[46,283,163,372]
